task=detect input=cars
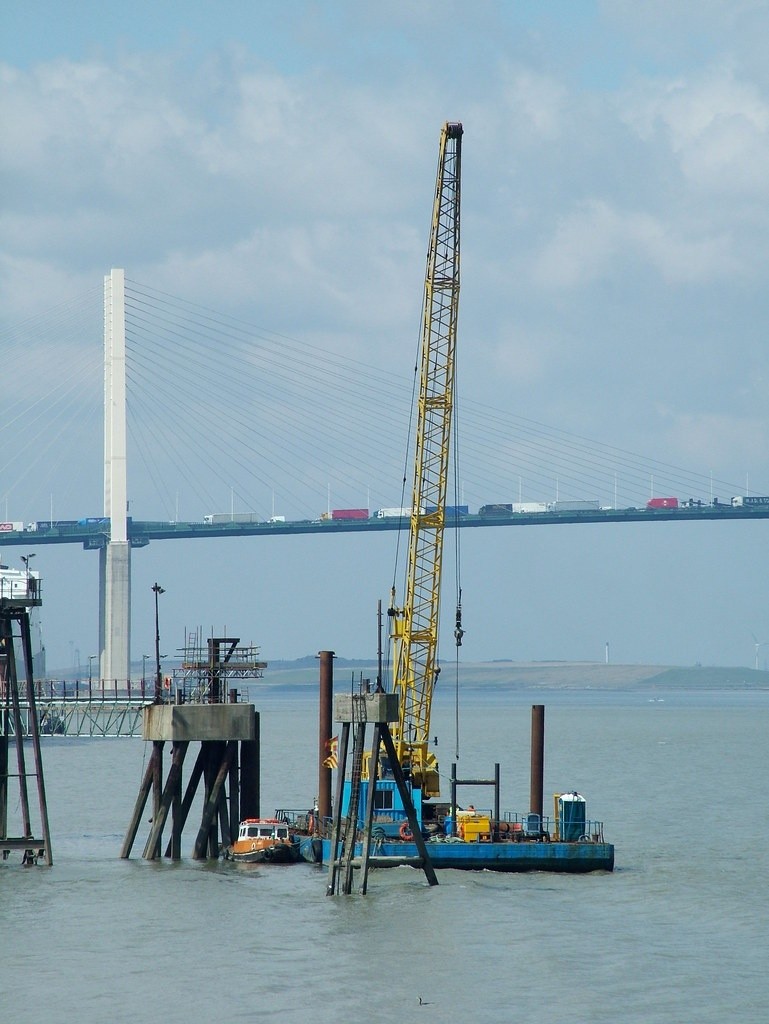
[624,507,637,512]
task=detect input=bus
[732,495,769,508]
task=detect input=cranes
[357,117,469,798]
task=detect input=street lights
[88,655,97,701]
[142,653,151,701]
[150,580,167,704]
[20,551,36,598]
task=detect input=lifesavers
[245,819,261,823]
[165,677,171,689]
[307,815,314,833]
[264,819,278,823]
[399,823,415,841]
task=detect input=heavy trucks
[26,520,78,533]
[372,505,425,519]
[321,508,370,521]
[478,500,600,515]
[76,516,133,533]
[203,511,257,527]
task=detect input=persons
[444,810,452,834]
[468,805,474,810]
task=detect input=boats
[220,815,299,864]
[274,649,617,876]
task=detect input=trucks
[645,497,679,510]
[267,516,285,524]
